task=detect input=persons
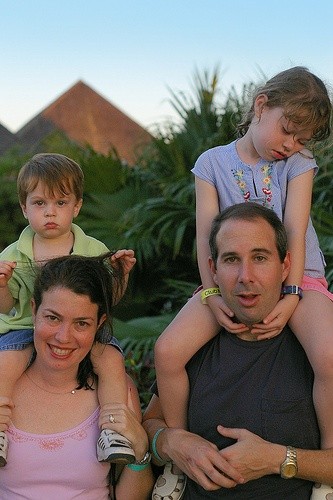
[141,203,333,500]
[151,66,333,500]
[0,255,154,500]
[0,153,136,467]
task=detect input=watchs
[281,282,303,301]
[135,450,151,465]
[280,445,298,480]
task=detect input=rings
[109,414,115,423]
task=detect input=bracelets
[200,288,221,305]
[126,462,149,471]
[152,427,170,464]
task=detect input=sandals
[151,461,188,500]
[309,482,333,500]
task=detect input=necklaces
[25,371,80,395]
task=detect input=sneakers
[0,432,9,467]
[96,429,137,464]
